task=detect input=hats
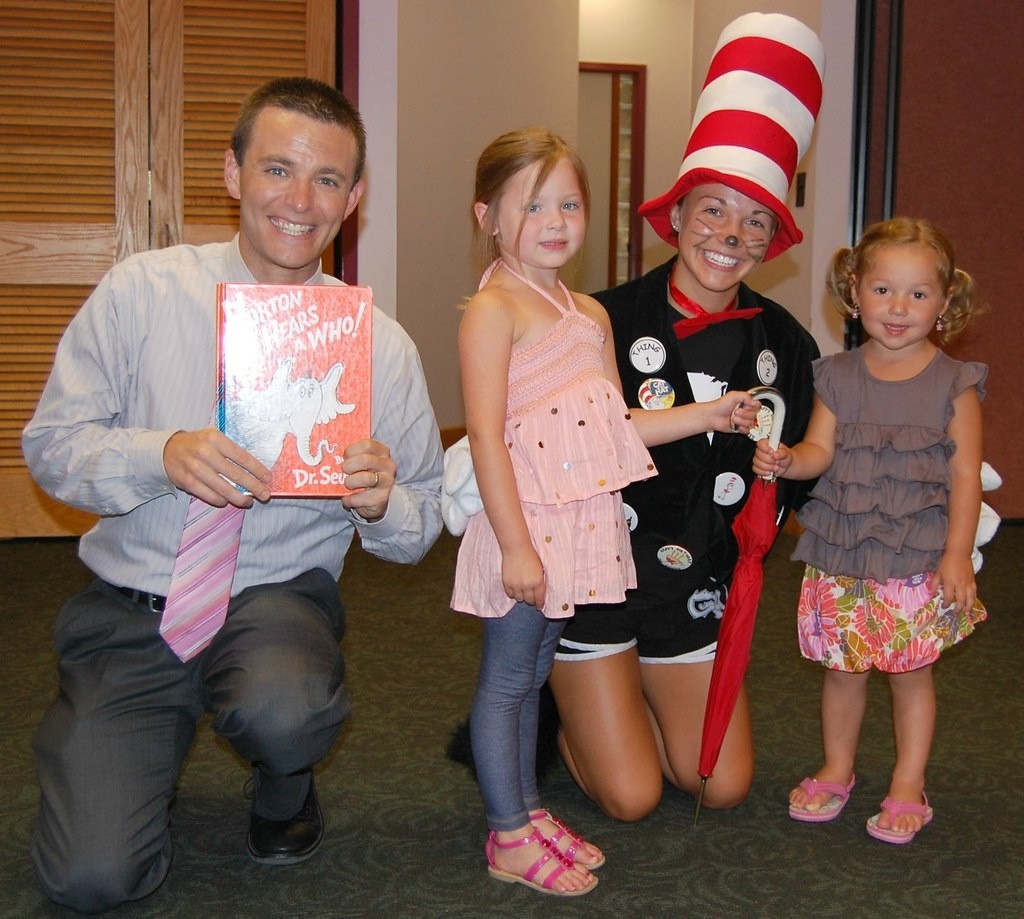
[637,12,825,263]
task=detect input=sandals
[486,827,600,897]
[867,791,933,843]
[789,772,856,822]
[530,811,607,869]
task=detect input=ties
[157,402,247,666]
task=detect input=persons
[753,216,1002,844]
[19,75,444,913]
[450,130,661,898]
[442,167,1002,820]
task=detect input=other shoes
[536,680,561,740]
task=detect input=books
[214,283,375,496]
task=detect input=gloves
[971,461,1002,575]
[441,436,485,535]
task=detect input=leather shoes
[243,765,327,865]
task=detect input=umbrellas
[691,386,788,823]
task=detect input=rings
[371,470,379,487]
